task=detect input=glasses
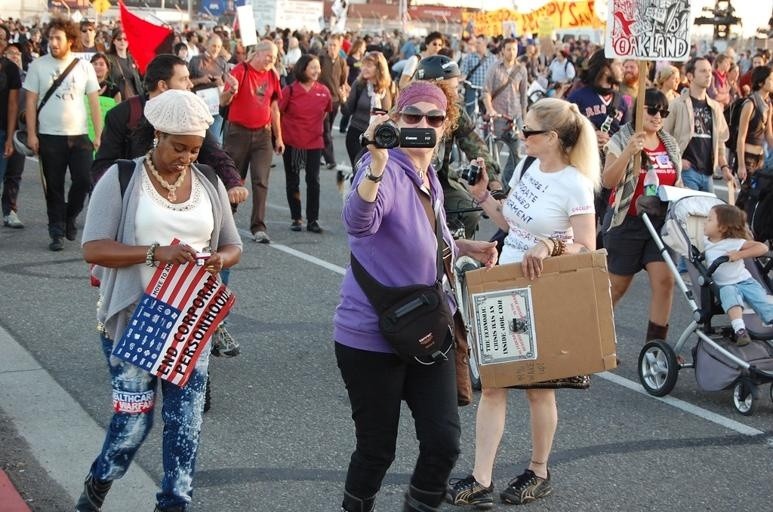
[82,28,92,32]
[398,106,446,127]
[643,107,668,117]
[115,37,127,40]
[521,126,545,137]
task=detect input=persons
[443,96,601,511]
[0,0,772,289]
[594,88,687,376]
[695,202,772,350]
[330,81,455,512]
[76,90,241,512]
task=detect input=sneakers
[735,329,750,346]
[210,324,240,358]
[442,476,493,508]
[499,470,551,504]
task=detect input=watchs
[362,165,385,187]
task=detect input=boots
[75,474,112,512]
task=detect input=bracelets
[228,90,237,96]
[539,236,570,259]
[473,194,493,207]
[144,242,173,271]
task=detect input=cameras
[459,164,482,186]
[193,253,211,266]
[373,119,436,151]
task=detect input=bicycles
[474,114,520,194]
[462,82,483,118]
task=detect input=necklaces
[410,164,429,182]
[146,149,192,204]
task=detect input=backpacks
[218,61,248,119]
[734,168,772,243]
[723,98,754,149]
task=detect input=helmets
[13,129,35,157]
[501,125,519,144]
[415,55,460,80]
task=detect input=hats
[143,89,214,138]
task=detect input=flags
[112,237,237,388]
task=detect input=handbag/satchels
[452,309,472,406]
[379,284,454,370]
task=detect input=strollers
[635,185,773,416]
